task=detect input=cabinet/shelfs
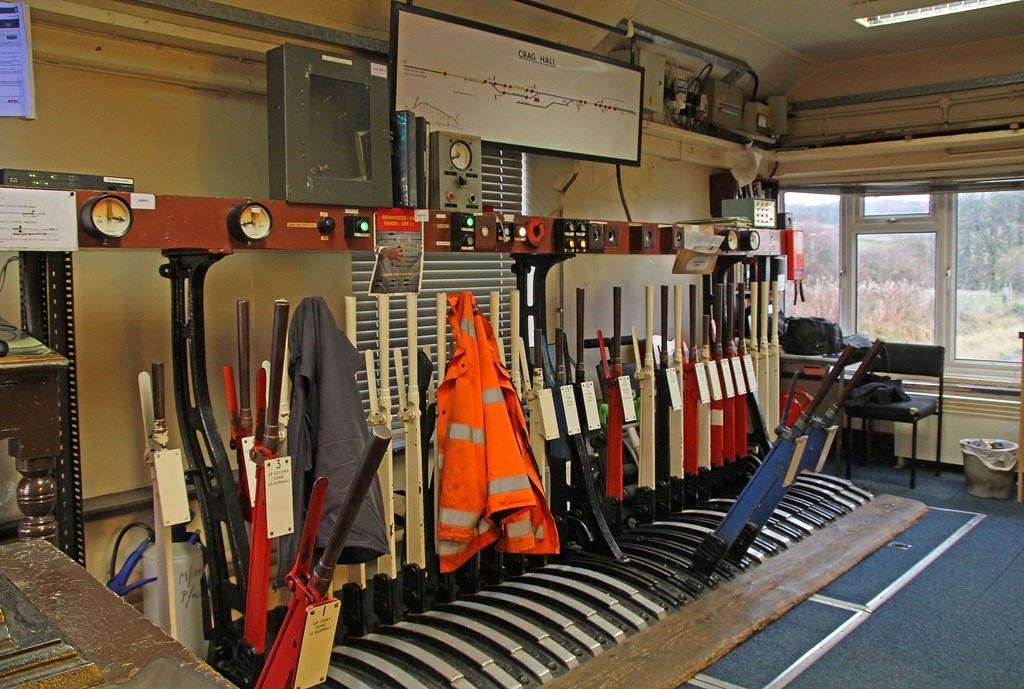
[709,173,779,296]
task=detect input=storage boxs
[264,40,394,209]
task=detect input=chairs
[843,340,946,490]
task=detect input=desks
[0,316,70,551]
[0,537,241,689]
[779,346,873,478]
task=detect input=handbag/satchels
[781,317,843,356]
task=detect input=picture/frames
[387,0,646,167]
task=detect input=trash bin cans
[958,437,1020,500]
